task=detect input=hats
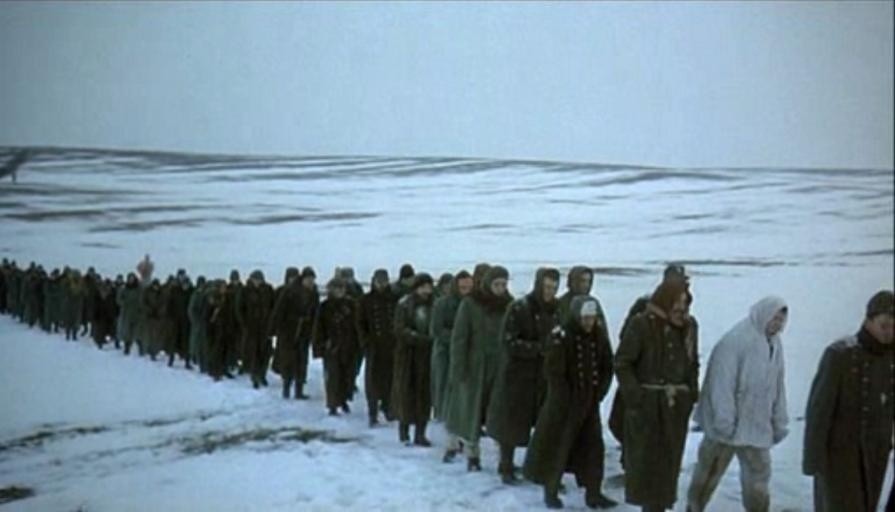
[580,301,597,317]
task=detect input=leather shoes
[504,474,521,485]
[399,426,410,446]
[498,462,524,473]
[586,494,618,508]
[545,496,563,508]
[416,432,431,445]
[443,449,455,461]
[468,457,480,471]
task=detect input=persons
[1,258,365,415]
[354,262,617,512]
[608,265,698,512]
[800,288,895,511]
[682,293,792,511]
